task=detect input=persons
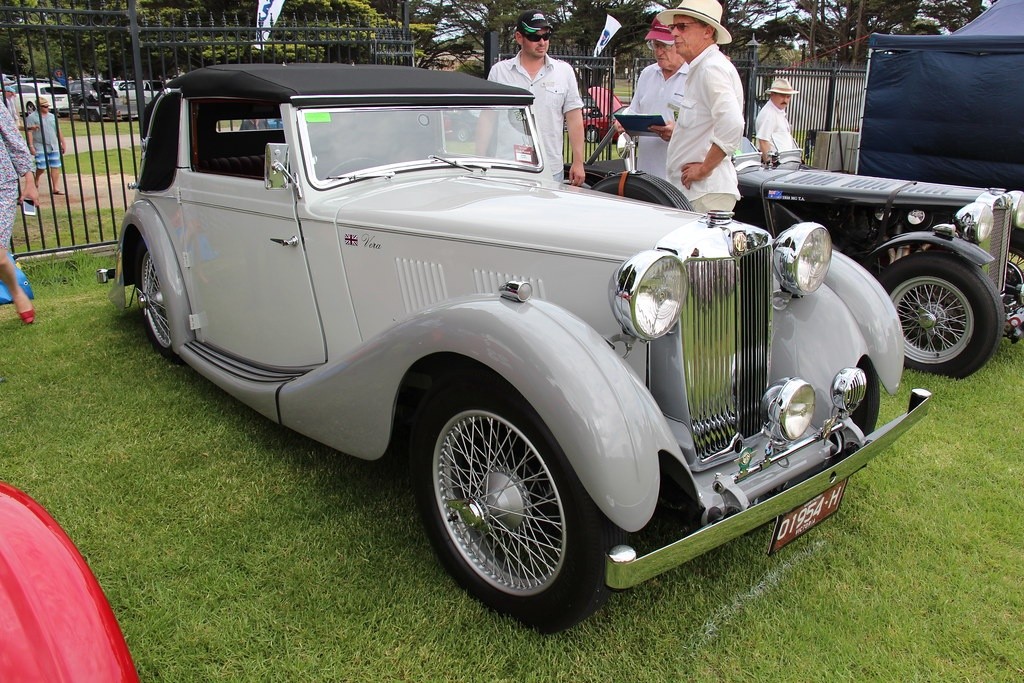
[753,77,803,167]
[486,7,587,191]
[610,0,748,217]
[0,75,67,326]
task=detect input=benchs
[198,154,265,180]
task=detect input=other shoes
[19,309,35,323]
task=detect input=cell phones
[23,198,36,216]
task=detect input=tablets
[614,113,666,137]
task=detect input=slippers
[52,190,64,195]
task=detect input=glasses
[646,39,674,51]
[39,105,49,108]
[519,30,550,42]
[668,20,708,33]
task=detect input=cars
[0,482,145,683]
[0,73,161,119]
[576,86,629,144]
[100,64,931,632]
[566,115,1024,377]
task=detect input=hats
[656,0,732,44]
[4,86,16,93]
[36,97,50,106]
[645,9,680,40]
[3,78,16,85]
[517,9,554,35]
[765,80,800,95]
